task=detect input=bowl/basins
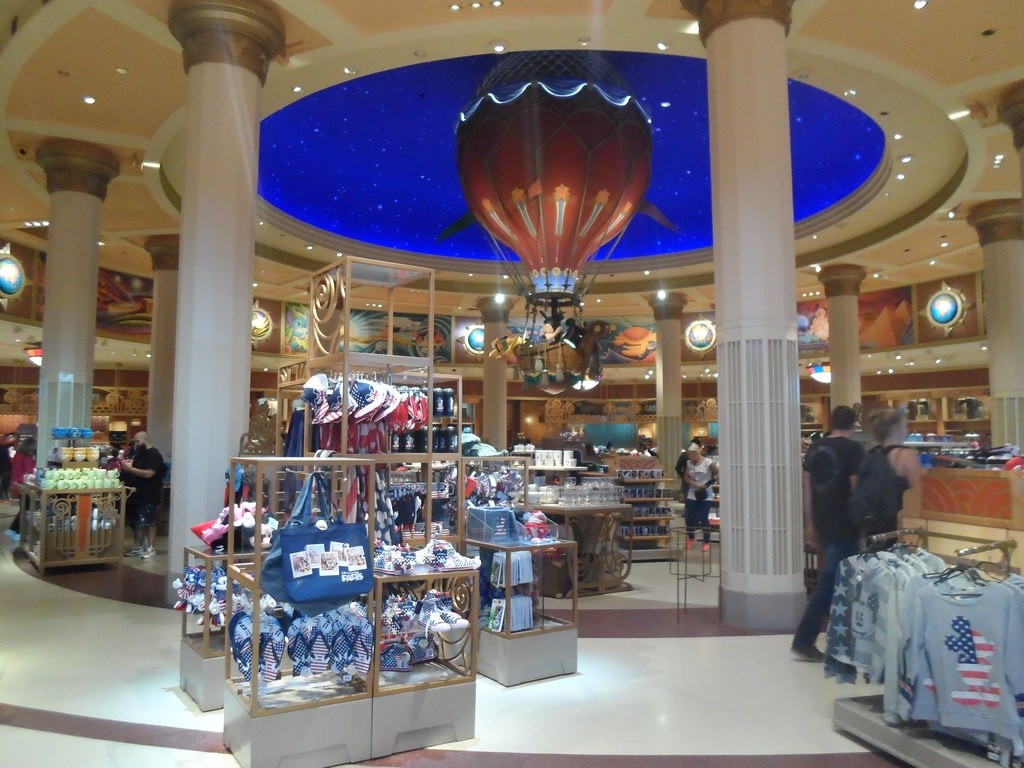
[47,446,100,462]
[52,427,93,437]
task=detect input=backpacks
[852,444,906,519]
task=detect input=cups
[402,521,450,539]
[33,467,122,489]
[415,481,456,498]
[509,444,624,505]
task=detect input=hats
[302,374,419,427]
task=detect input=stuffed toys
[207,502,273,552]
[172,565,241,626]
[538,309,564,340]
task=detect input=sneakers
[141,547,157,558]
[124,545,144,557]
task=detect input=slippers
[172,564,376,686]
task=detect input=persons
[121,431,164,559]
[6,437,38,541]
[676,438,719,551]
[790,405,920,661]
[639,441,651,457]
[107,448,122,471]
[0,433,16,501]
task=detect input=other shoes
[377,536,483,673]
[687,539,694,549]
[702,544,710,551]
[791,641,824,663]
[5,529,21,540]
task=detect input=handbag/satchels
[695,488,707,500]
[260,472,375,616]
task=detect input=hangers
[923,544,1010,596]
[856,529,918,561]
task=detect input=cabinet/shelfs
[182,255,722,768]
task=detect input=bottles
[391,386,457,452]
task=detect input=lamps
[806,361,831,384]
[22,343,42,367]
[564,327,581,349]
[571,374,598,391]
